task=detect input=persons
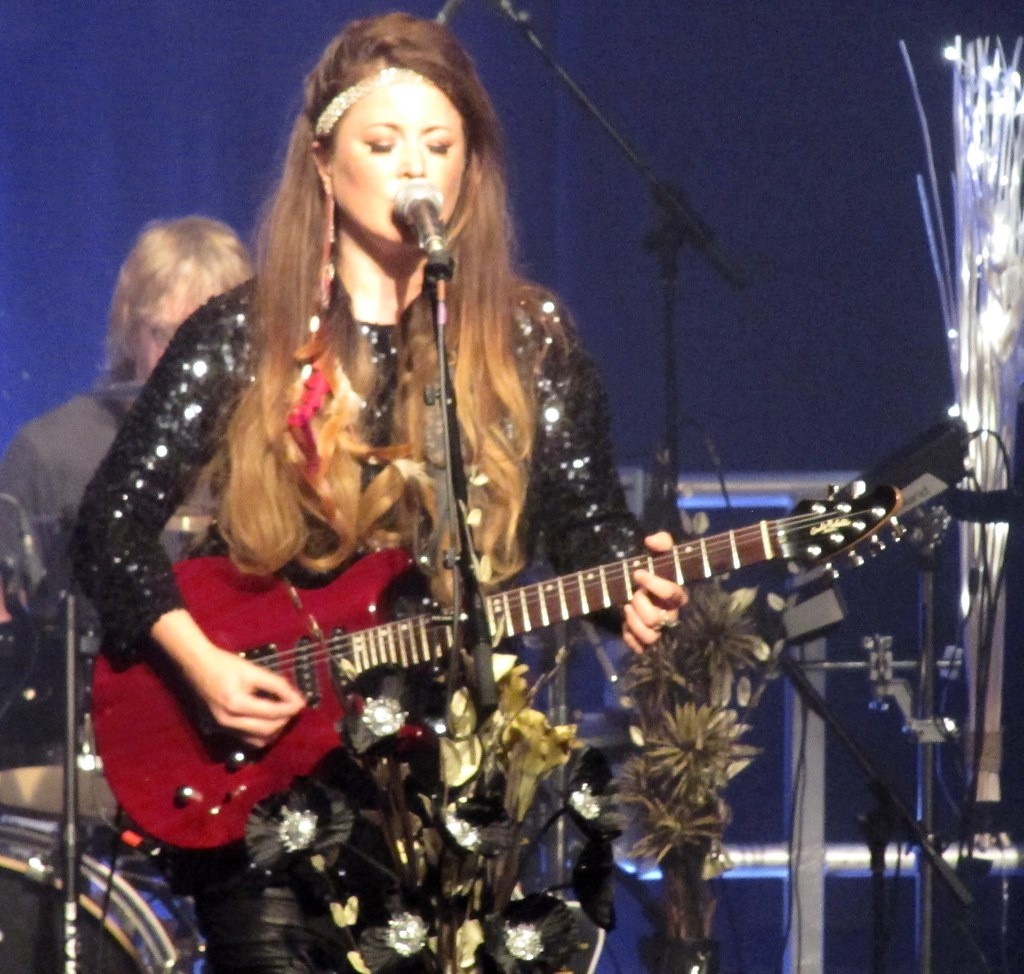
[71,12,689,974]
[1,214,253,631]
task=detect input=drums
[0,763,195,974]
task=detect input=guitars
[85,470,909,857]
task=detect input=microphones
[436,0,463,26]
[392,185,452,276]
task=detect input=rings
[655,617,671,630]
